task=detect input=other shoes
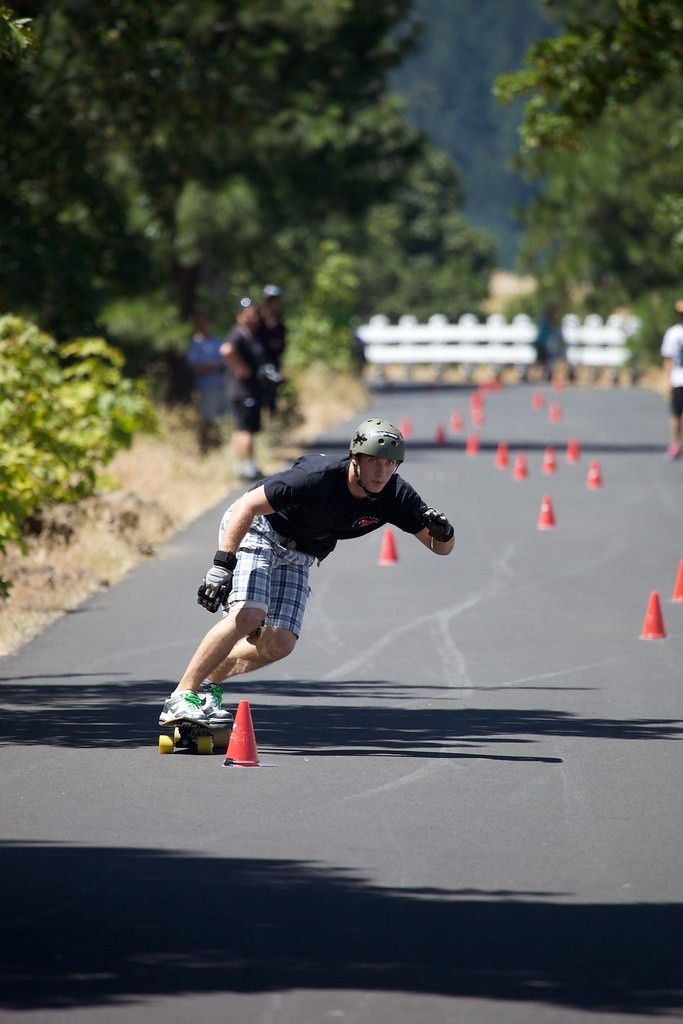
[663,443,683,464]
[236,471,266,482]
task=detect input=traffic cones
[494,441,508,473]
[449,407,465,437]
[535,495,558,530]
[532,390,547,409]
[401,414,413,437]
[224,699,260,767]
[466,430,479,456]
[434,423,447,444]
[549,402,562,423]
[549,375,566,394]
[567,439,581,466]
[640,590,669,639]
[510,451,531,483]
[468,376,503,426]
[377,528,400,565]
[585,458,605,487]
[670,558,683,603]
[542,441,557,477]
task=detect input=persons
[254,283,287,425]
[157,419,455,722]
[183,322,229,456]
[216,297,266,482]
[533,301,565,381]
[659,297,683,463]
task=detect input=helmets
[348,417,407,464]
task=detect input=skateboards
[158,718,232,753]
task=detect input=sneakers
[158,691,210,727]
[196,682,235,724]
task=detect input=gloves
[196,550,238,613]
[416,505,455,543]
